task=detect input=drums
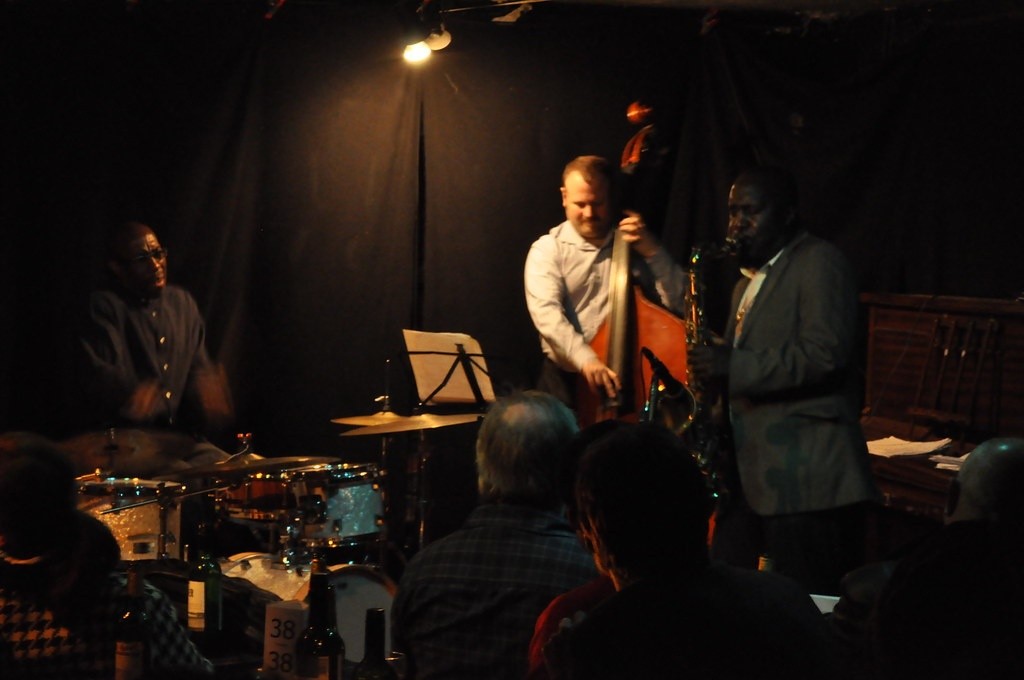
[284,458,386,539]
[207,469,327,522]
[215,551,396,661]
[75,473,186,563]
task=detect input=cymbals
[151,452,343,479]
[341,411,480,436]
[60,430,198,474]
[330,410,403,426]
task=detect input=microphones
[641,347,694,421]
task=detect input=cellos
[574,96,717,544]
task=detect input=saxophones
[643,234,749,497]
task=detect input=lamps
[426,17,452,51]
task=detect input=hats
[573,416,710,551]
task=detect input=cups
[383,650,406,678]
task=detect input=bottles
[112,569,153,680]
[186,525,224,659]
[353,607,398,680]
[294,573,345,680]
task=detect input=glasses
[125,247,168,268]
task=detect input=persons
[0,432,220,680]
[829,437,1024,680]
[544,427,830,680]
[528,419,624,680]
[76,221,235,463]
[389,390,598,680]
[524,156,687,425]
[687,164,870,600]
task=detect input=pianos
[853,292,1024,517]
